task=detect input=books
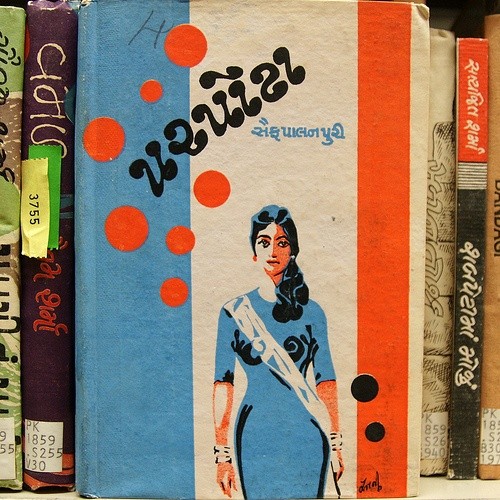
[0,5,25,491]
[479,14,500,481]
[421,27,456,477]
[446,37,491,479]
[73,0,432,499]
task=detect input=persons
[22,1,79,492]
